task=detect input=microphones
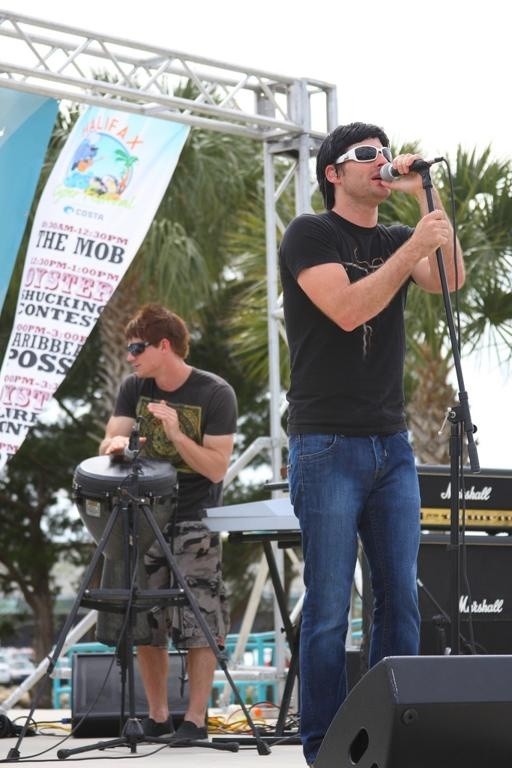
[380,156,443,183]
[124,421,141,460]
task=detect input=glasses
[127,341,146,355]
[333,143,394,165]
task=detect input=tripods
[57,641,239,760]
[7,460,272,760]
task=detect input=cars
[0,647,34,687]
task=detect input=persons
[99,302,240,748]
[280,122,467,768]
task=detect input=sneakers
[170,720,209,740]
[139,715,175,737]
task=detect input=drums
[72,455,179,648]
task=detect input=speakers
[70,652,207,738]
[361,536,512,670]
[311,655,512,768]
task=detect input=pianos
[201,472,512,533]
